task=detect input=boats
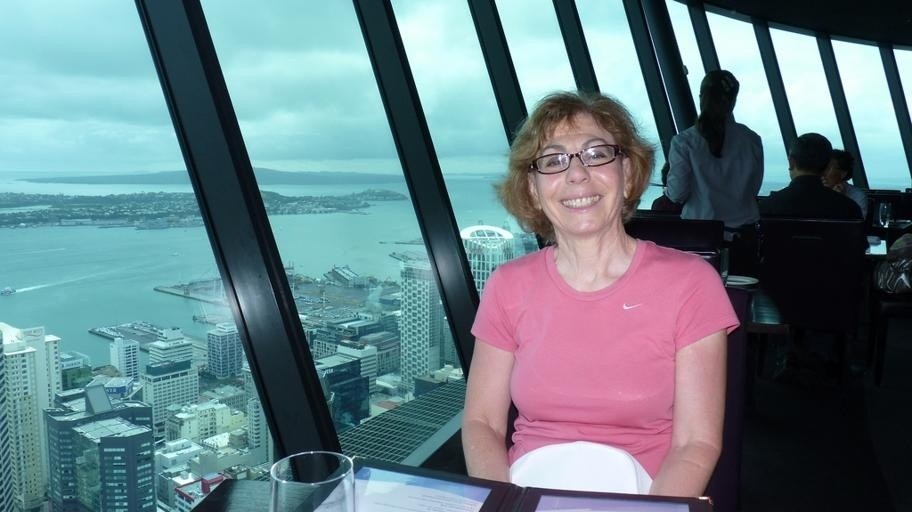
[1,287,16,295]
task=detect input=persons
[651,161,684,211]
[459,84,742,498]
[662,69,764,260]
[822,149,867,218]
[757,133,867,219]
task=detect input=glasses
[527,142,628,175]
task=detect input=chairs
[623,186,911,440]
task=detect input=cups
[879,203,891,228]
[269,451,356,512]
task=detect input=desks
[191,477,714,511]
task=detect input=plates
[726,275,758,287]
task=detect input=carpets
[739,358,894,510]
